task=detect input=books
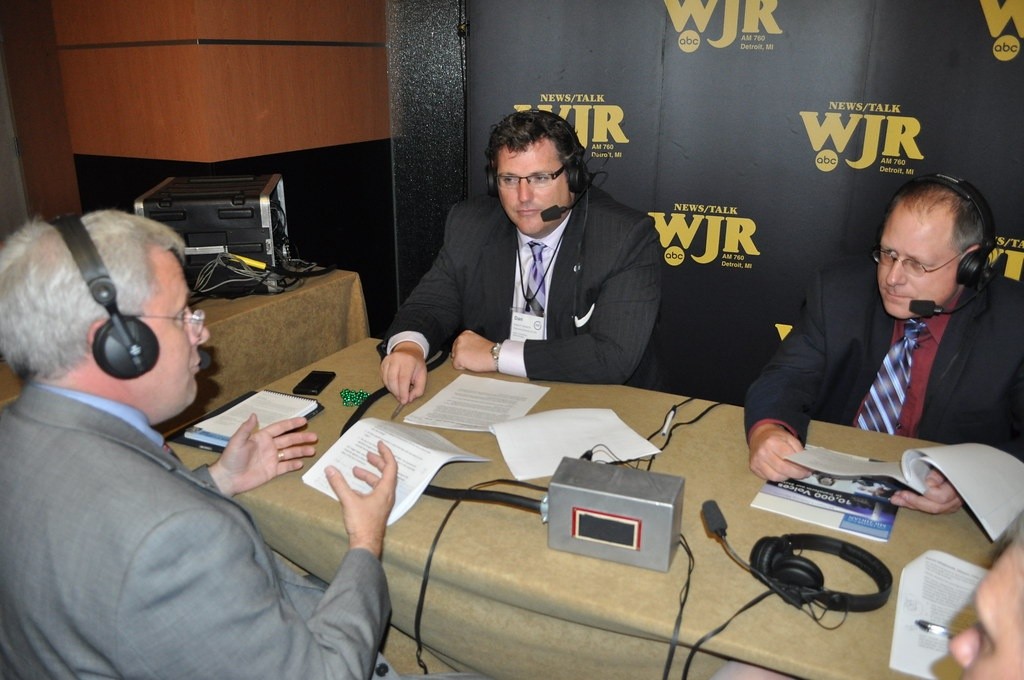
[167,388,324,453]
[750,443,907,542]
[784,442,1023,544]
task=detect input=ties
[855,319,927,435]
[523,239,549,318]
[160,440,185,465]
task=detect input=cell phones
[293,370,336,396]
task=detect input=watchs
[490,342,502,372]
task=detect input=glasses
[872,240,973,278]
[133,308,206,338]
[494,165,566,189]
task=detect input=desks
[0,269,371,434]
[162,337,996,680]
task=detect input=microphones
[540,178,594,222]
[909,284,989,317]
[703,500,800,606]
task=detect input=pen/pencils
[390,386,413,420]
[916,620,952,637]
[661,404,677,437]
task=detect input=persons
[376,110,666,405]
[814,472,897,497]
[0,210,398,680]
[948,521,1024,680]
[744,175,1024,514]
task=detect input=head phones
[486,109,586,197]
[749,533,893,612]
[872,173,996,284]
[51,212,159,380]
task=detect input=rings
[450,354,453,359]
[278,450,285,462]
